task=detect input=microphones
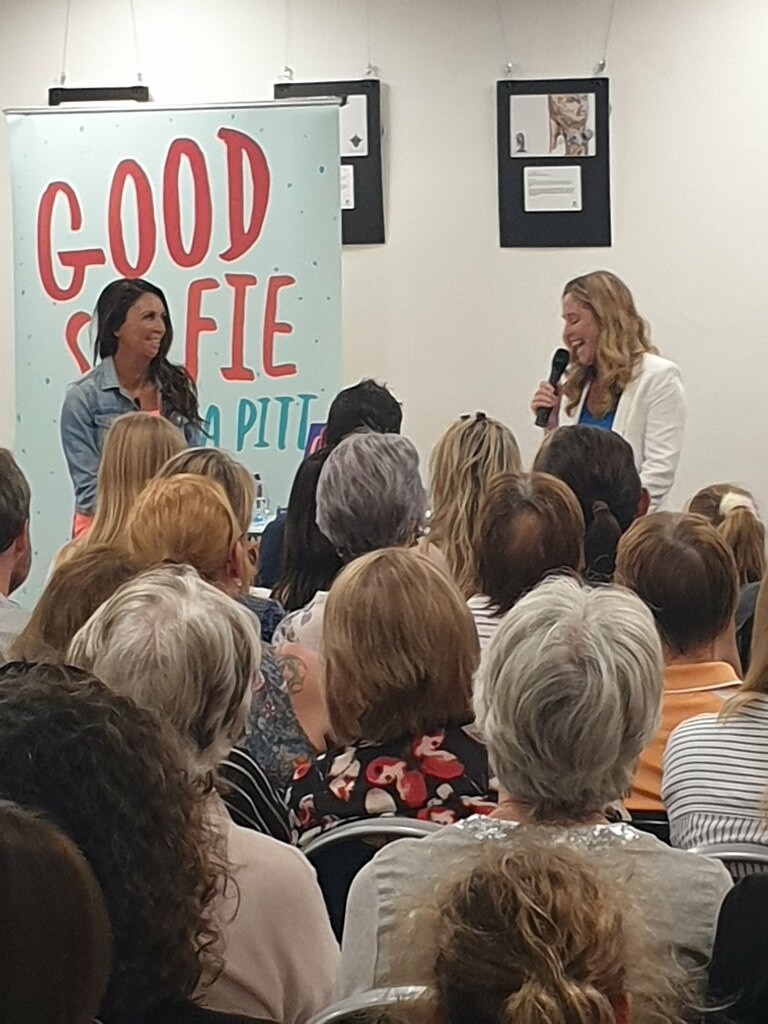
[535,348,571,428]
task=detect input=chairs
[284,810,450,1024]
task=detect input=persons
[532,270,687,514]
[0,381,768,1024]
[61,280,203,544]
